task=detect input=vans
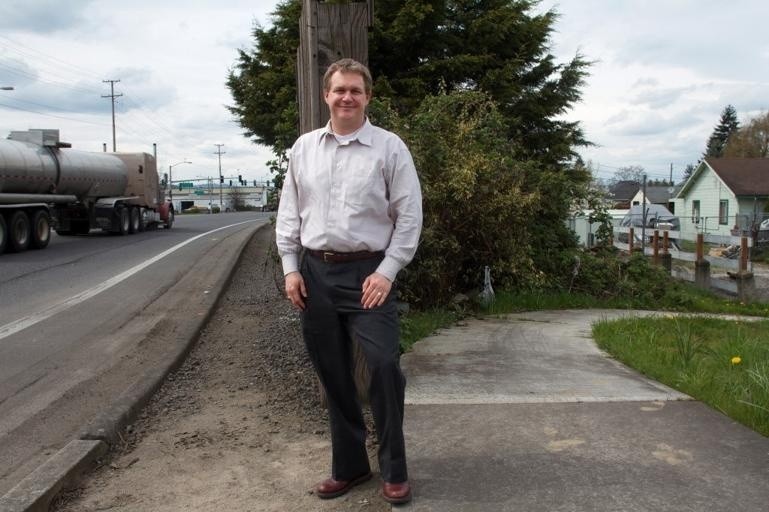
[619,204,680,246]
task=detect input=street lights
[0,85,14,90]
[169,161,192,198]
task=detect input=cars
[212,203,231,212]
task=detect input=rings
[378,292,382,295]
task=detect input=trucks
[0,130,174,253]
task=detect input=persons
[275,58,424,505]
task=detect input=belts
[306,249,383,263]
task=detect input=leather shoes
[382,479,412,504]
[317,471,373,499]
